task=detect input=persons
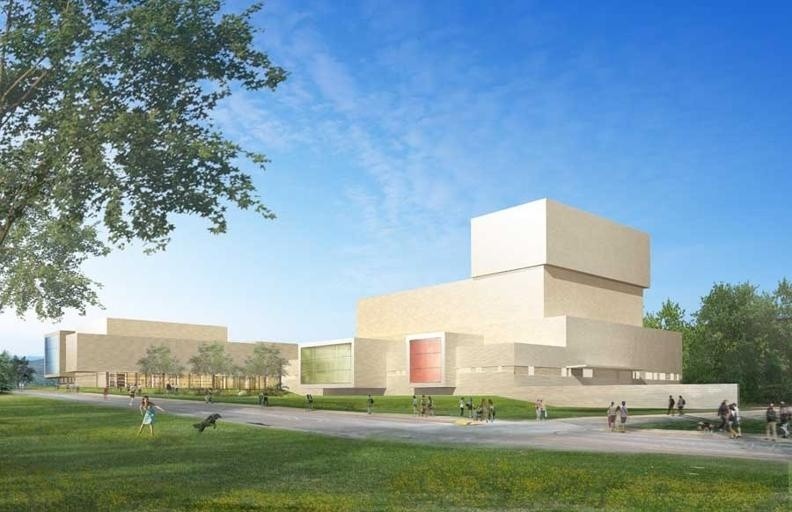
[607,402,616,432]
[727,404,739,439]
[305,394,310,409]
[620,401,628,432]
[127,387,139,407]
[104,383,111,400]
[667,395,675,416]
[532,398,541,423]
[136,395,166,438]
[615,406,622,432]
[763,402,778,441]
[412,394,433,419]
[779,400,790,438]
[205,387,214,405]
[126,382,142,394]
[66,382,80,394]
[732,403,742,437]
[367,393,372,415]
[257,389,264,406]
[458,396,497,423]
[717,400,729,431]
[538,397,548,423]
[262,390,269,407]
[677,395,686,415]
[309,394,314,410]
